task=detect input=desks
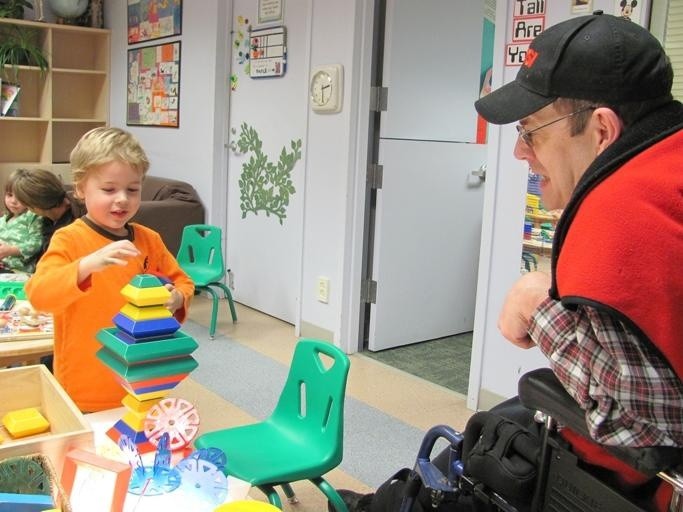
[38,402,265,511]
[0,271,54,367]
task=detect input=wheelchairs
[369,368,683,512]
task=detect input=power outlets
[315,275,330,303]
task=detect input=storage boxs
[0,362,96,477]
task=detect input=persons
[0,168,42,275]
[25,125,195,415]
[13,167,84,273]
[472,8,683,512]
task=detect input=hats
[474,9,673,125]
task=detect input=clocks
[306,62,345,115]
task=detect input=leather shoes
[327,490,363,511]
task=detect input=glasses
[516,104,599,147]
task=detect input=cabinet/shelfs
[0,17,112,166]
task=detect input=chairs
[174,223,238,340]
[193,337,350,511]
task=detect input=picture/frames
[125,0,183,45]
[123,39,181,128]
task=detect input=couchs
[59,173,206,262]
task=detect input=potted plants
[0,23,53,103]
[0,0,34,22]
